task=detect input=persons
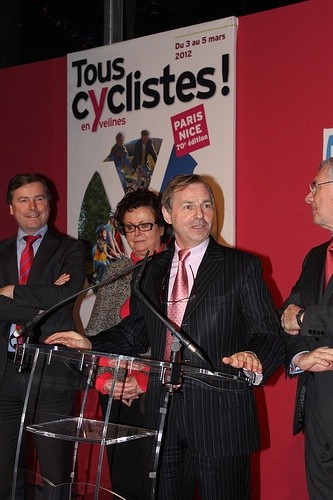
[83,187,172,500]
[110,128,158,191]
[275,157,333,500]
[89,207,127,287]
[0,173,87,500]
[44,174,287,500]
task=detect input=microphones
[12,250,206,363]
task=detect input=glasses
[308,180,333,195]
[121,221,158,234]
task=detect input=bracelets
[296,307,306,329]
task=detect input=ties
[161,249,190,390]
[15,234,42,345]
[323,236,333,292]
